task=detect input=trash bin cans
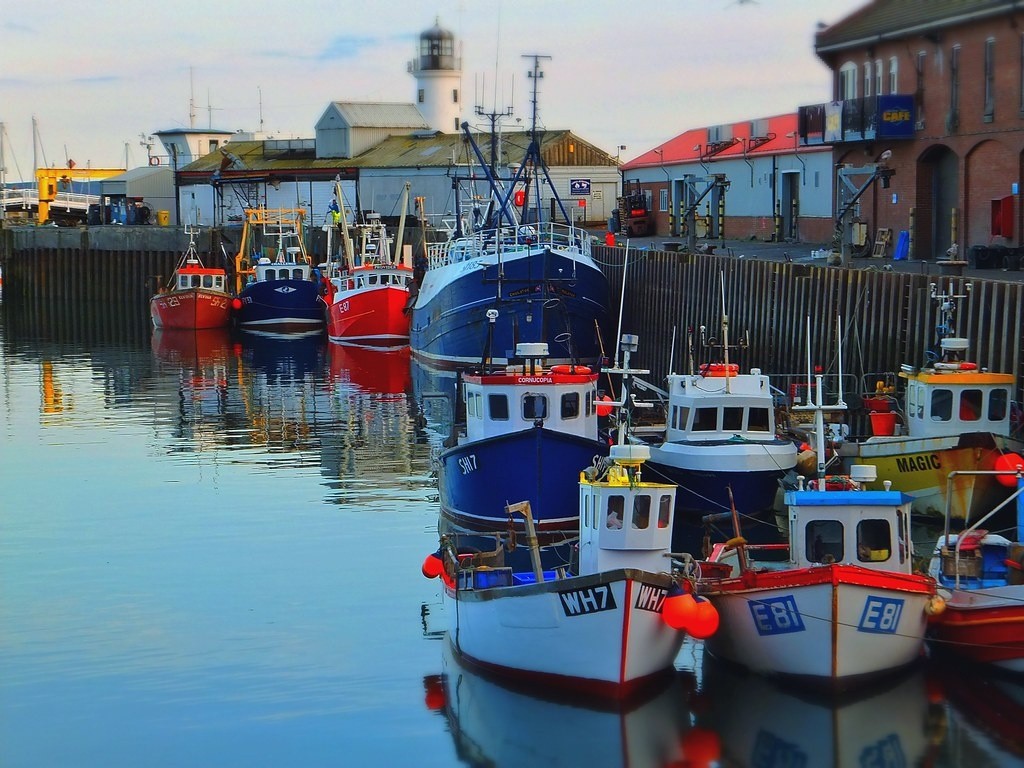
[89,205,102,225]
[937,261,964,275]
[49,206,88,225]
[157,210,170,228]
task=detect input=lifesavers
[933,362,977,370]
[699,363,739,377]
[550,365,592,375]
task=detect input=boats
[233,204,327,341]
[145,218,231,328]
[318,174,420,352]
[402,49,614,379]
[421,259,1024,694]
